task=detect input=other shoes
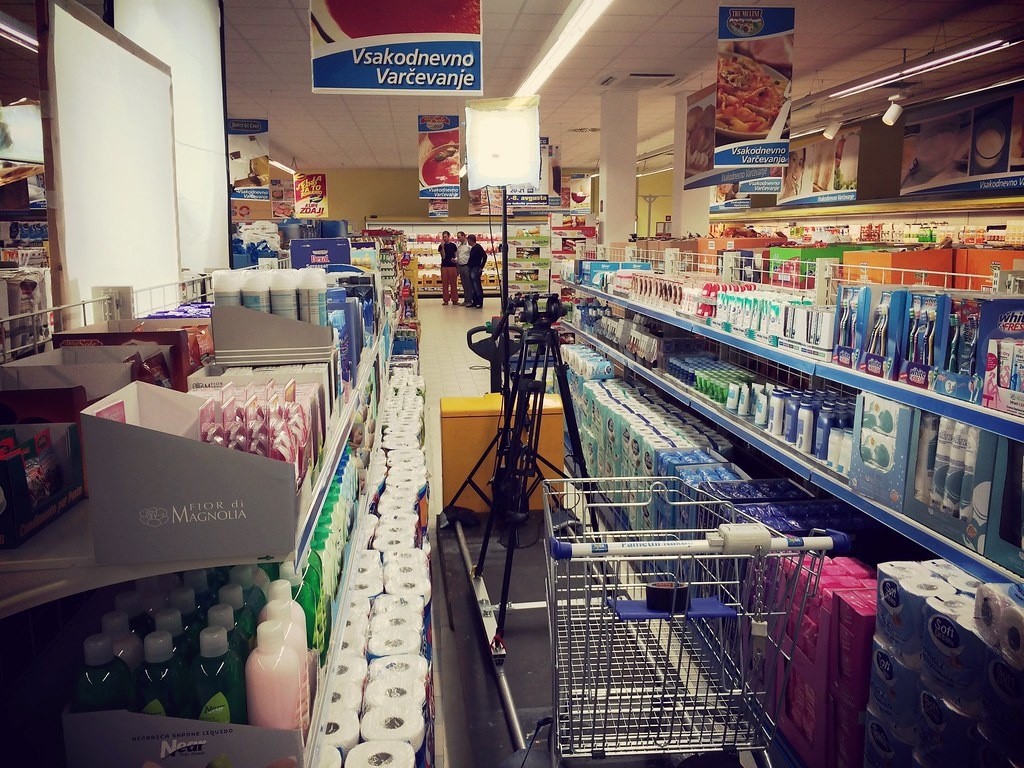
[453,301,461,305]
[461,301,472,307]
[442,301,448,305]
[470,303,482,308]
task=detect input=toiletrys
[661,347,983,528]
[57,235,386,768]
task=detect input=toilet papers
[862,556,1024,768]
[560,342,734,537]
[307,367,432,768]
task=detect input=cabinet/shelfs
[561,190,1024,768]
[365,213,586,299]
[0,256,395,768]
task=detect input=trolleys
[535,472,852,767]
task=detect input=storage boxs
[0,213,1024,768]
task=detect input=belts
[459,264,468,266]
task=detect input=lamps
[269,0,1024,178]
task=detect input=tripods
[444,289,613,647]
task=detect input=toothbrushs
[832,284,1024,419]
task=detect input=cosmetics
[681,283,812,337]
[76,451,358,748]
[667,353,856,474]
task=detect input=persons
[18,279,41,325]
[467,234,487,308]
[457,232,472,307]
[778,147,806,201]
[716,183,738,202]
[438,231,459,305]
